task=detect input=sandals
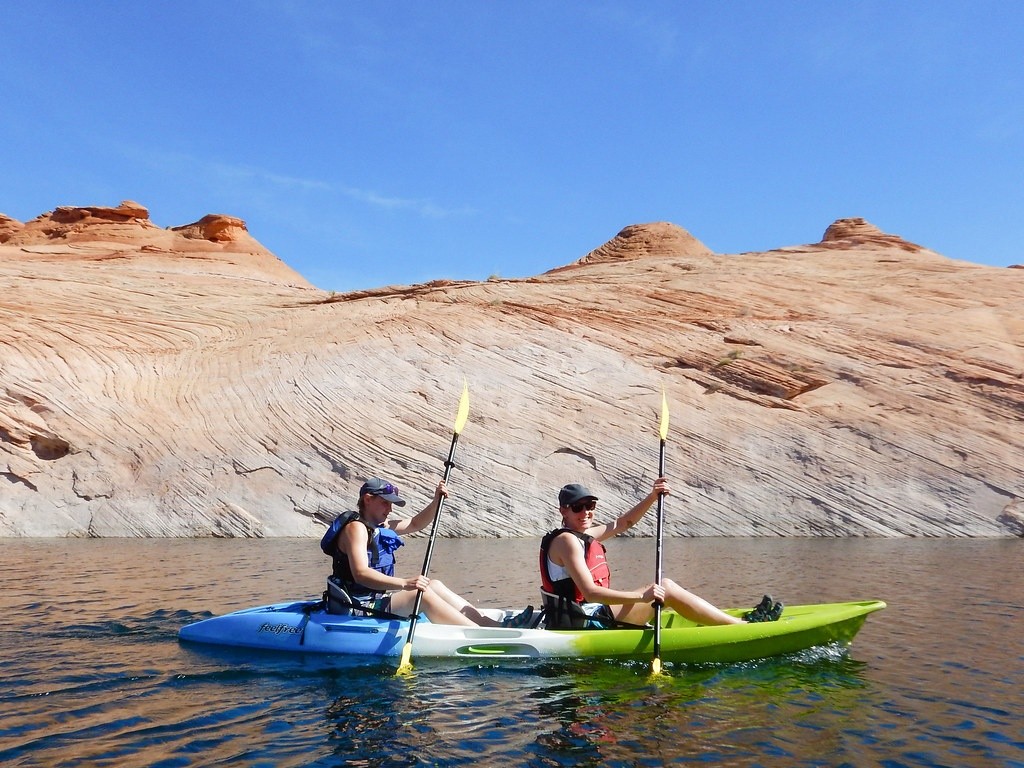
[502,605,534,627]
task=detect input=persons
[320,478,534,628]
[540,477,784,630]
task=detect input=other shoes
[744,595,772,618]
[749,601,784,623]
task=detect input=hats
[361,477,406,507]
[559,484,598,506]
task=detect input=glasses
[382,485,399,495]
[573,501,597,512]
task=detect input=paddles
[395,379,468,674]
[652,381,669,675]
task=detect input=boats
[177,595,887,670]
